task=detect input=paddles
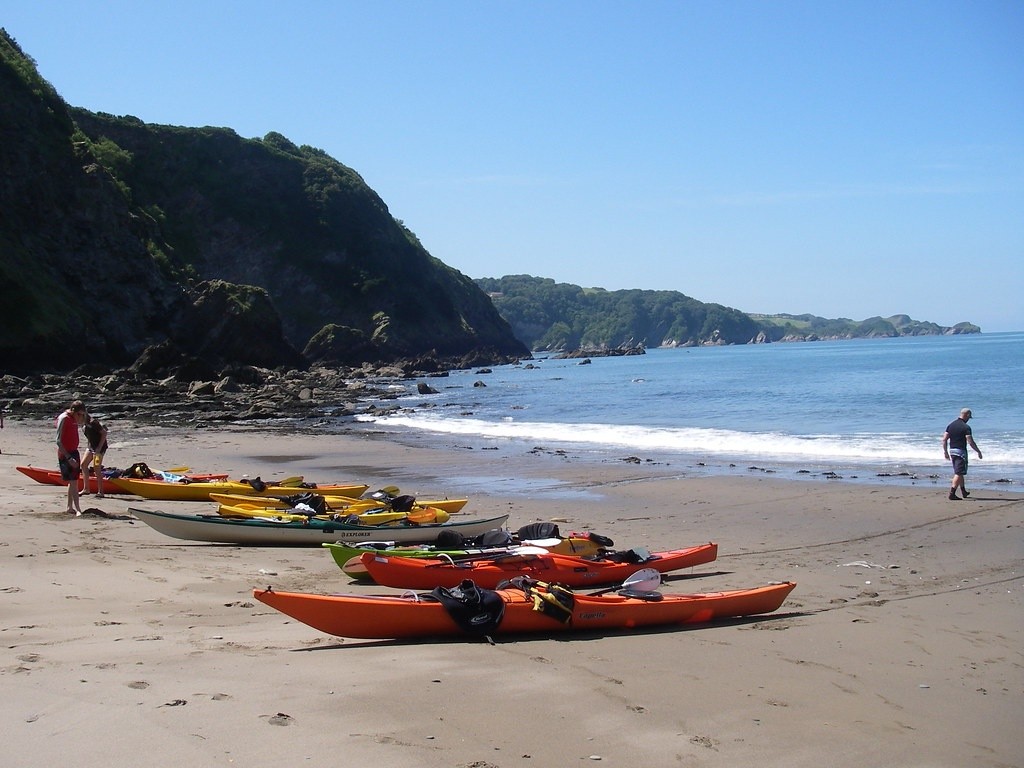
[196,514,292,524]
[428,537,562,551]
[425,545,550,568]
[382,485,400,496]
[583,567,661,596]
[165,467,189,473]
[269,477,303,487]
[375,509,437,527]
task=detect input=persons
[54,400,88,517]
[78,412,108,498]
[941,408,983,501]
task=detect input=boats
[15,465,469,523]
[360,541,718,589]
[322,522,608,581]
[253,576,798,640]
[128,507,510,546]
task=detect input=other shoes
[78,489,89,496]
[96,491,104,497]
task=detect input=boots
[961,488,970,497]
[949,488,962,500]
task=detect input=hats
[961,409,973,418]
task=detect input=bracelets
[66,456,73,461]
